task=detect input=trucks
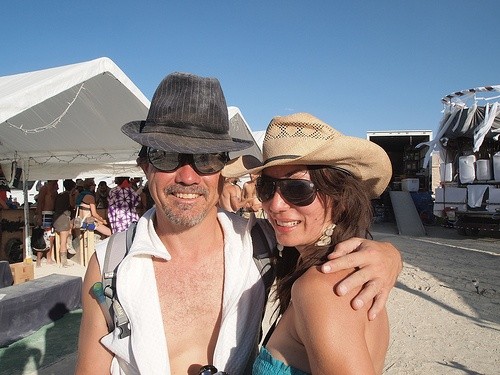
[365,130,434,225]
[431,85,500,239]
[6,160,148,210]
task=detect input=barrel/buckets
[476,159,493,180]
[392,182,401,191]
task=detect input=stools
[33,247,49,267]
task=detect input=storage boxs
[9,262,34,284]
[401,177,420,192]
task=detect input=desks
[0,206,37,261]
[0,273,82,348]
[20,226,54,261]
[454,210,500,239]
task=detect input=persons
[28,176,155,268]
[220,173,269,221]
[222,111,393,375]
[74,72,402,375]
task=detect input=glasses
[255,174,320,206]
[89,184,97,187]
[147,147,230,176]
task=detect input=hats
[120,73,254,153]
[220,113,392,199]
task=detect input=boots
[59,252,74,267]
[66,234,77,254]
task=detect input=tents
[226,106,267,169]
[0,57,150,260]
